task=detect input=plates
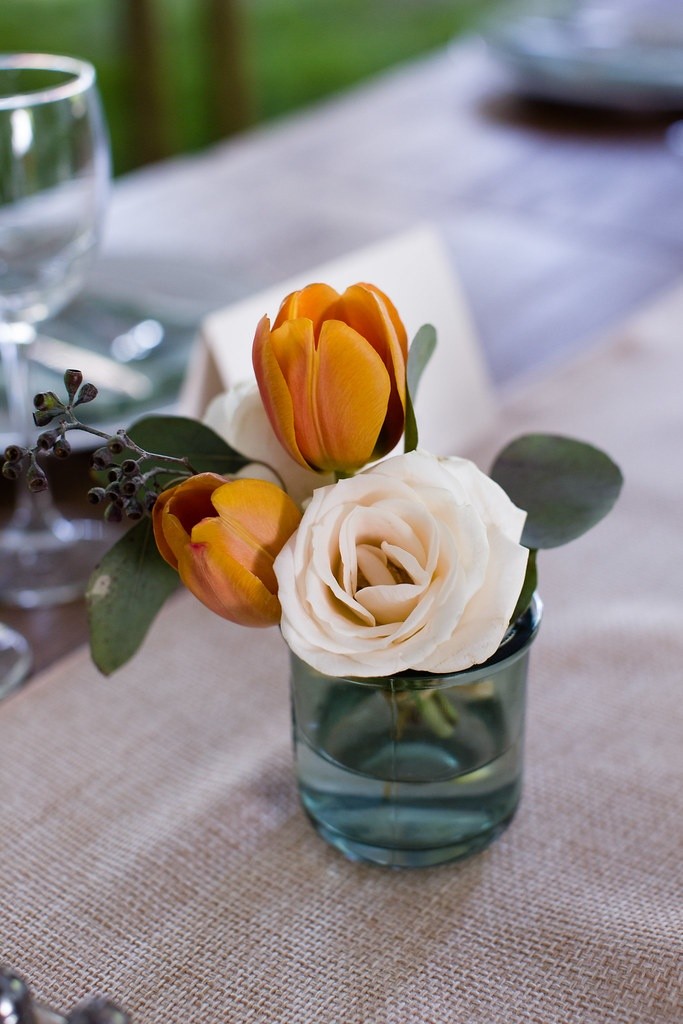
[492,1,683,112]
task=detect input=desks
[0,0,683,1024]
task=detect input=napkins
[181,226,490,484]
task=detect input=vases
[289,593,542,874]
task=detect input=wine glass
[0,49,138,612]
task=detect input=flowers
[0,283,627,739]
